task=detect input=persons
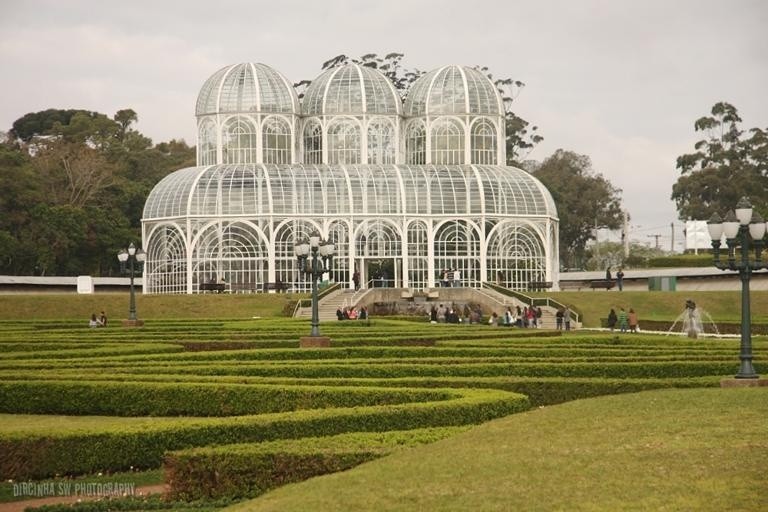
[368,266,394,288]
[439,267,461,287]
[335,301,572,331]
[626,308,638,333]
[605,266,612,291]
[618,307,627,331]
[208,277,216,293]
[351,269,360,291]
[99,311,107,326]
[88,313,104,328]
[607,308,618,331]
[218,277,227,293]
[615,267,625,290]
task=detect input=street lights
[115,241,148,321]
[294,228,337,338]
[706,196,768,382]
